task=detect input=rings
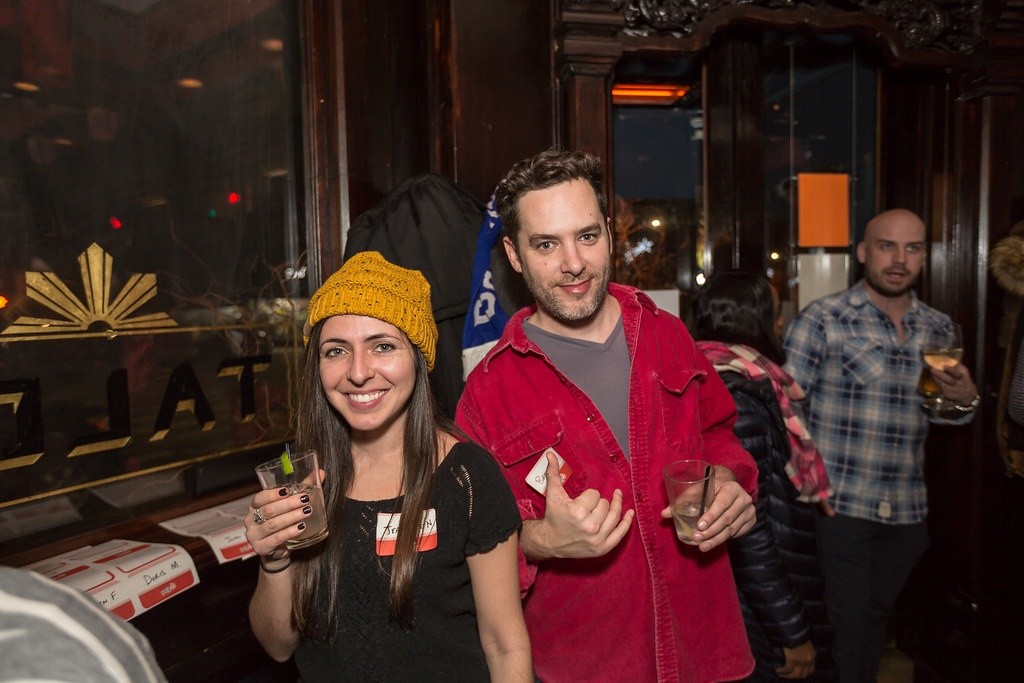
[253,510,265,524]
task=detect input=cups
[257,448,331,551]
[662,461,715,545]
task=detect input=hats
[302,251,438,372]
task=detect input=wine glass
[922,321,964,411]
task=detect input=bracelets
[259,558,291,573]
[954,394,980,412]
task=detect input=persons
[779,204,981,683]
[694,270,839,682]
[988,222,1024,478]
[456,153,761,683]
[244,251,534,683]
